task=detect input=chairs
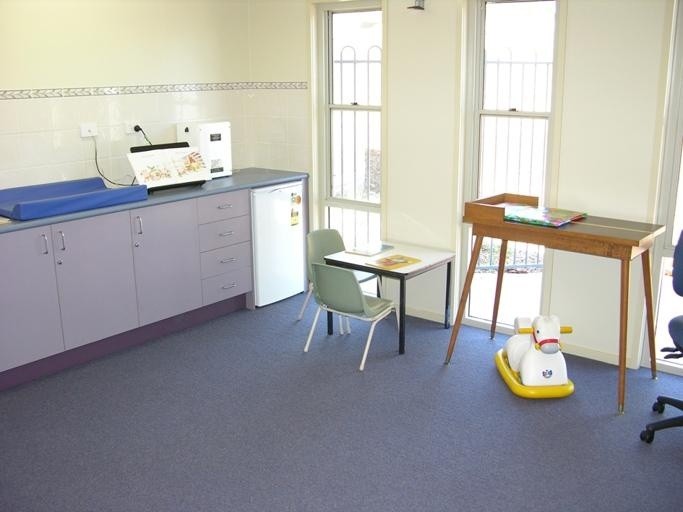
[298,228,400,371]
[638,230,683,443]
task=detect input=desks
[322,242,457,354]
[443,193,668,413]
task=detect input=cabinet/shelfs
[131,199,203,348]
[1,209,140,372]
[198,190,252,324]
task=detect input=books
[366,254,421,272]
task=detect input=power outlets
[125,120,140,135]
[80,121,98,138]
